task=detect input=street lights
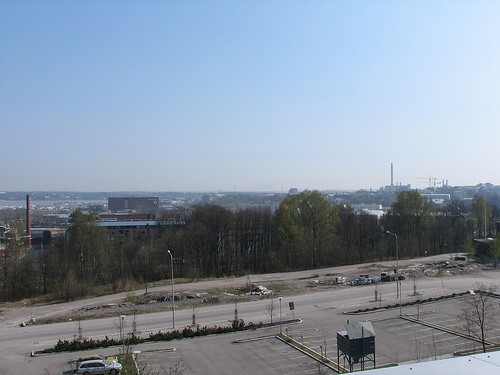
[386,231,399,298]
[167,250,175,328]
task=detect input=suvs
[76,360,122,375]
[76,354,118,368]
